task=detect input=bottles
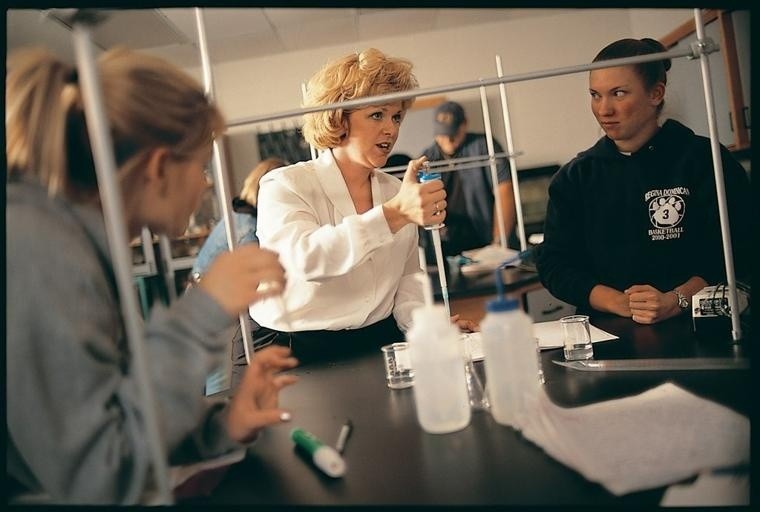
[419,423,483,508]
[479,298,543,426]
[404,304,473,435]
[463,330,488,410]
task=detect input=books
[390,314,624,374]
[512,378,750,496]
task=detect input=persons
[7,45,302,504]
[182,156,294,398]
[535,38,759,338]
[229,47,482,405]
[414,98,515,257]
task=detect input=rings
[435,202,440,213]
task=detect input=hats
[433,101,465,135]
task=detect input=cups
[388,388,415,430]
[566,361,600,403]
[531,337,546,387]
[380,341,413,390]
[558,313,594,362]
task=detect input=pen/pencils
[291,427,346,479]
[336,418,353,453]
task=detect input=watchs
[670,289,689,315]
